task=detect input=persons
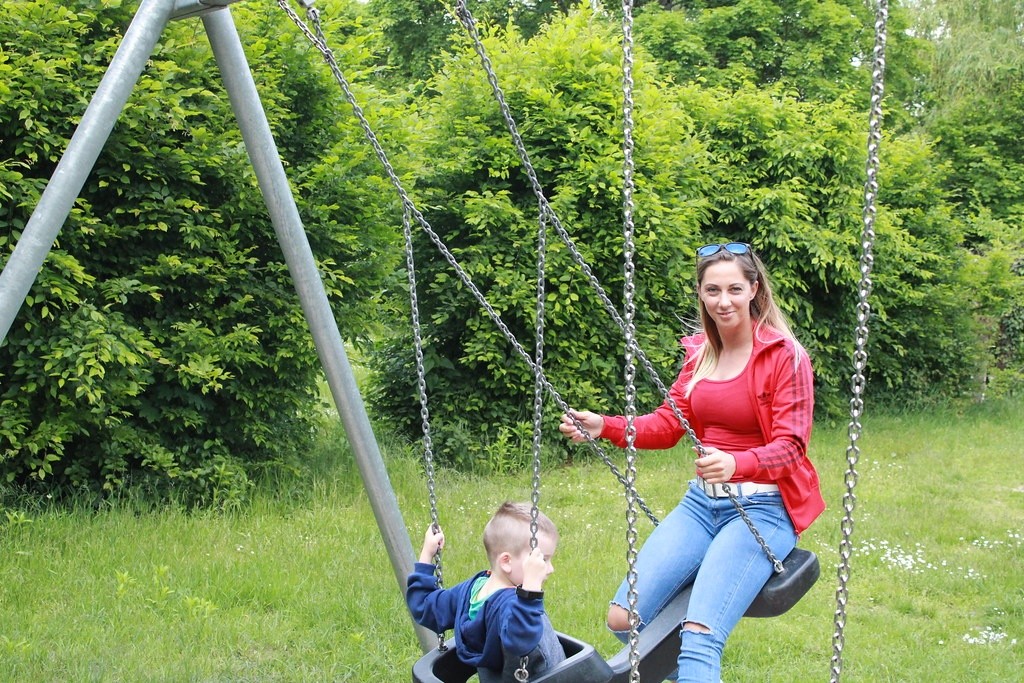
[407,500,566,683]
[559,242,826,683]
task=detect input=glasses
[696,241,753,282]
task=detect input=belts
[696,475,778,497]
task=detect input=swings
[274,1,821,683]
[619,1,891,683]
